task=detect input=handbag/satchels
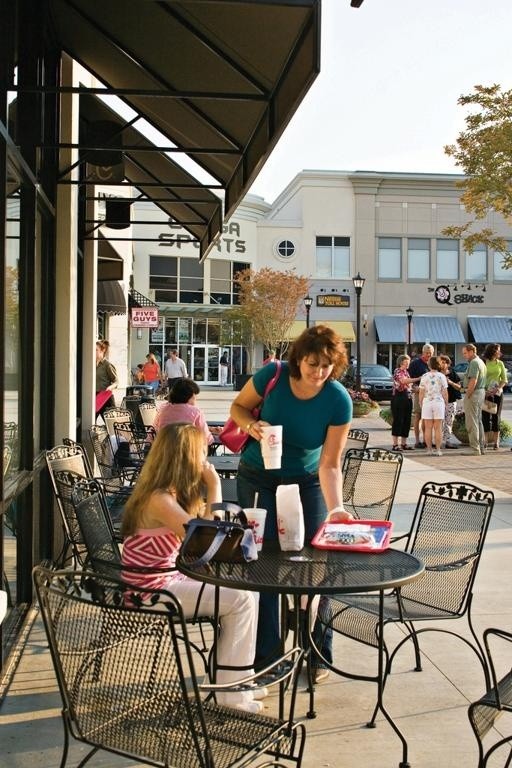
[177,499,260,570]
[219,357,282,455]
[481,398,498,414]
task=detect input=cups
[242,506,268,552]
[259,425,285,472]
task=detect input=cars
[451,362,511,392]
[343,365,395,399]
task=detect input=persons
[219,351,228,386]
[143,353,160,396]
[152,377,215,446]
[133,364,144,385]
[164,348,189,392]
[263,350,276,366]
[390,343,509,456]
[231,325,354,689]
[121,422,269,715]
[96,340,118,426]
[144,400,169,457]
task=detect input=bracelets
[247,420,257,434]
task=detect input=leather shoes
[309,660,333,685]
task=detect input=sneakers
[433,449,443,457]
[425,450,434,456]
[460,449,481,456]
[222,702,262,718]
[250,684,268,700]
[425,442,436,448]
[484,444,489,449]
[446,444,457,449]
[415,442,426,449]
[494,442,499,450]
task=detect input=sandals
[402,444,415,450]
[392,444,403,451]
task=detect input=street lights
[352,272,366,393]
[405,306,413,355]
[304,294,313,329]
